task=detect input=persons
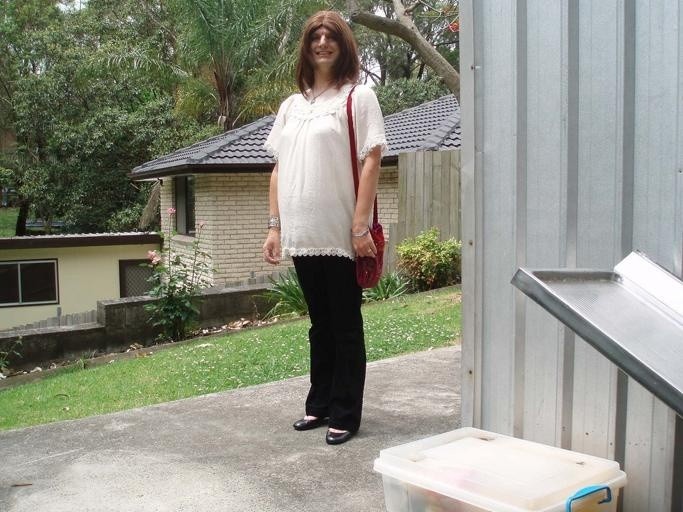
[263,10,388,445]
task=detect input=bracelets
[352,228,370,239]
[267,217,281,230]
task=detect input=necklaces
[311,87,330,104]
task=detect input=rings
[367,249,371,252]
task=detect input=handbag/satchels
[355,223,385,288]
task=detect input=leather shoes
[293,416,324,430]
[326,428,352,444]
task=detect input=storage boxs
[372,425,631,512]
